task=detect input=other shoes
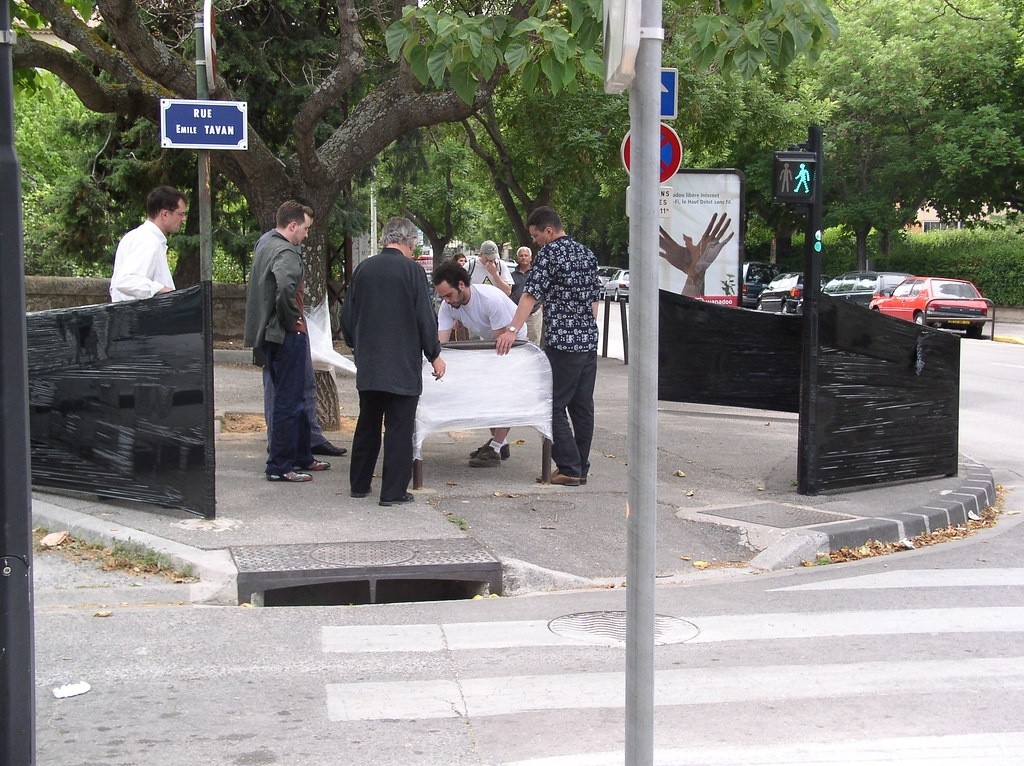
[294,459,330,471]
[312,441,348,455]
[266,469,312,481]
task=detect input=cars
[605,269,631,302]
[822,271,915,312]
[740,262,782,310]
[596,266,623,294]
[756,273,831,316]
[868,276,988,340]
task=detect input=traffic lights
[775,151,816,202]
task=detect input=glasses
[162,208,186,217]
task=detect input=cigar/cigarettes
[435,371,443,382]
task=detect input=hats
[480,240,500,260]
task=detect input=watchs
[507,325,519,334]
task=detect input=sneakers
[469,445,502,467]
[470,438,511,458]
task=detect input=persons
[340,217,446,506]
[243,200,331,482]
[430,259,527,468]
[495,207,601,486]
[109,186,188,303]
[452,253,467,267]
[463,240,515,297]
[263,197,347,457]
[507,246,543,348]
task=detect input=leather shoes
[536,469,586,485]
[379,491,414,506]
[350,486,373,497]
